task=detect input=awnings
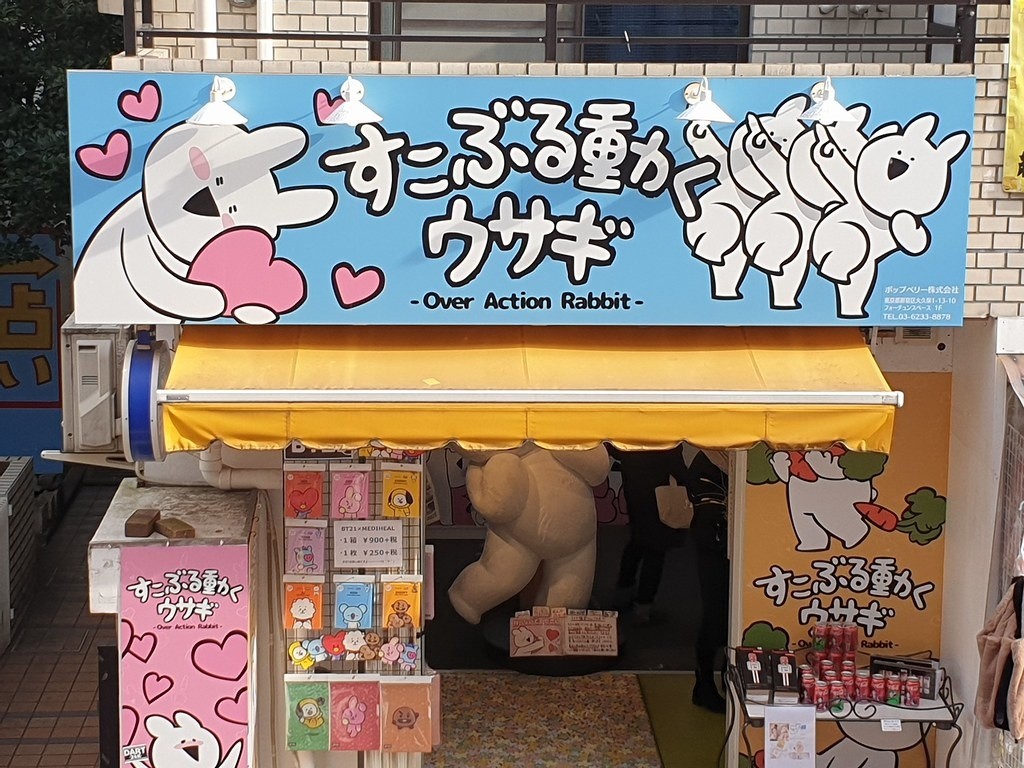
[157,324,904,454]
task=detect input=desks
[715,646,965,768]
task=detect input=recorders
[871,655,942,701]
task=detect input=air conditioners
[60,311,132,454]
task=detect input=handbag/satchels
[655,474,694,530]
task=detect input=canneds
[798,621,920,712]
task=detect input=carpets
[637,673,726,768]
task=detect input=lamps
[321,74,383,126]
[675,75,736,127]
[796,74,857,125]
[185,75,248,128]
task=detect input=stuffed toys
[448,440,610,626]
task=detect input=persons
[771,725,789,740]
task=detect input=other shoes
[630,609,666,626]
[607,583,638,608]
[692,685,726,715]
